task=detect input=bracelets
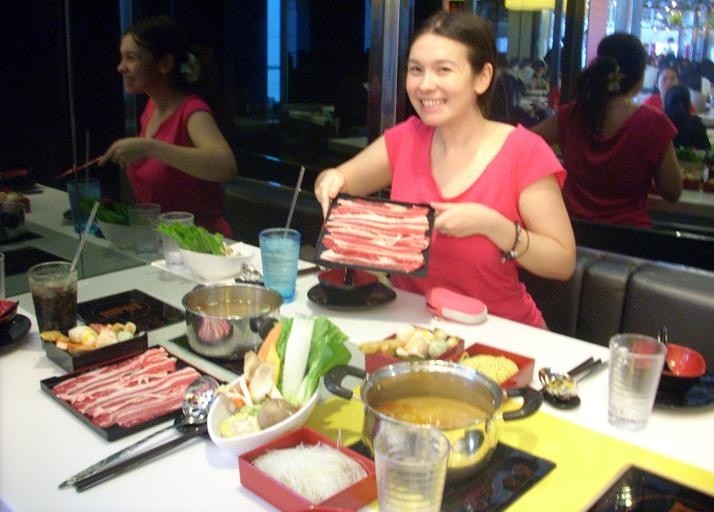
[500,221,531,263]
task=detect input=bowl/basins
[206,368,320,457]
[317,268,379,300]
[0,300,17,334]
[96,219,133,249]
[4,170,31,187]
[176,244,254,281]
[633,340,707,401]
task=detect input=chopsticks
[71,425,206,493]
[536,356,602,397]
[297,265,320,277]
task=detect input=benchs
[220,177,324,251]
[518,242,714,376]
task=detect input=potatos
[257,398,293,430]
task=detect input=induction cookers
[585,462,713,511]
[76,287,185,332]
[168,334,243,376]
[345,435,558,510]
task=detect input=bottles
[642,39,700,93]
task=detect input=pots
[324,358,542,484]
[179,281,285,362]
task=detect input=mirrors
[401,0,714,256]
[1,0,398,299]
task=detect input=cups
[258,227,300,303]
[67,178,101,235]
[608,331,668,431]
[375,425,451,512]
[0,252,5,301]
[28,261,77,350]
[158,210,194,268]
[128,203,161,258]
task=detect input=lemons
[219,414,259,439]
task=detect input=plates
[304,284,395,309]
[63,202,112,220]
[620,359,713,409]
[0,314,30,346]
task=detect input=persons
[313,10,577,331]
[97,15,237,240]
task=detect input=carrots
[257,322,283,363]
